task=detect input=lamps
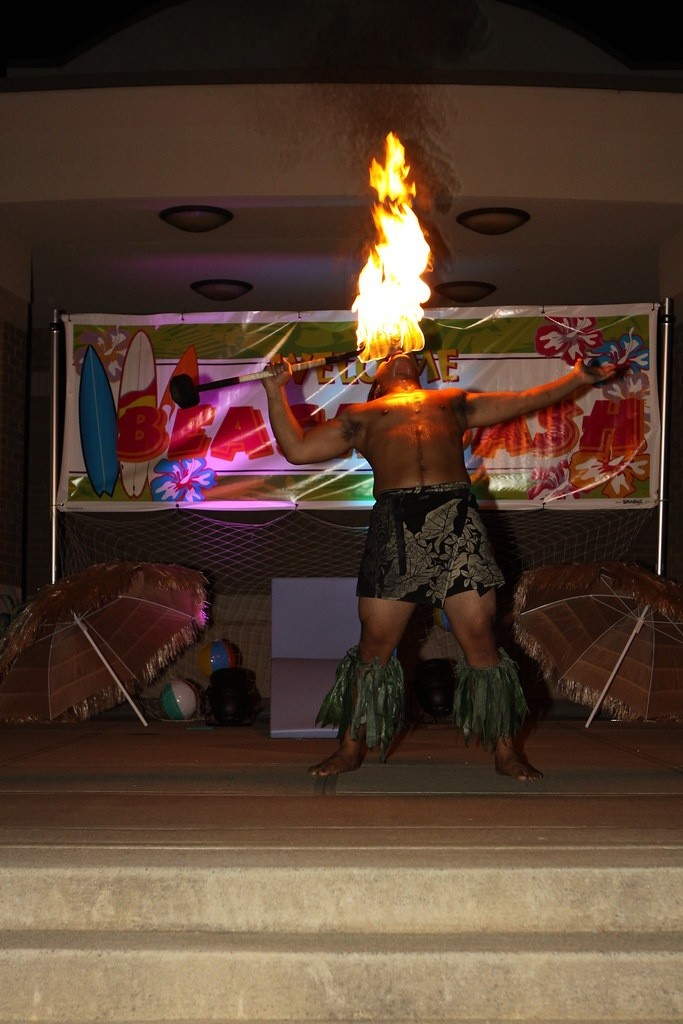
[435,281,494,303]
[158,204,233,234]
[456,208,531,234]
[191,280,253,302]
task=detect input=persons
[262,352,616,782]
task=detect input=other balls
[199,640,241,680]
[159,679,199,721]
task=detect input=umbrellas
[513,559,682,729]
[0,559,211,728]
